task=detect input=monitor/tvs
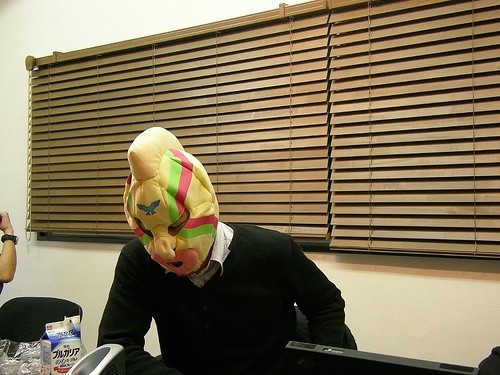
[285,340,480,375]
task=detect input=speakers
[65,343,127,374]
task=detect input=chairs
[0,297,84,358]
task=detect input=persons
[0,210,19,283]
[97,127,346,375]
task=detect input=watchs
[1,234,18,245]
